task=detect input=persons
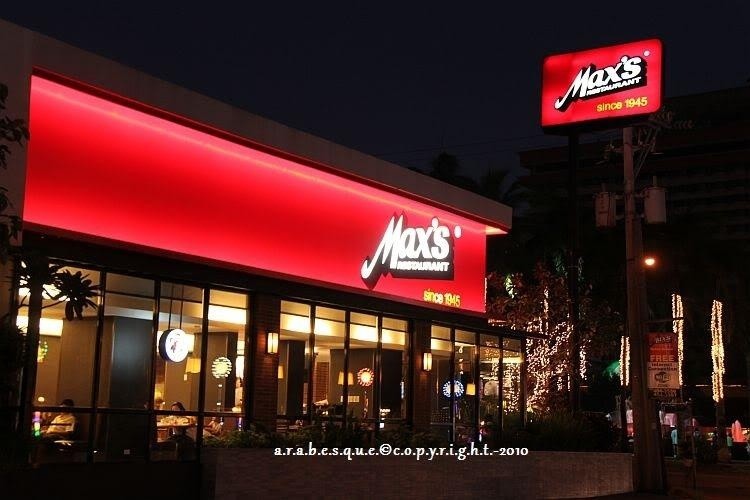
[727,433,734,455]
[171,401,199,441]
[208,410,223,433]
[713,432,718,447]
[34,398,78,463]
[693,428,701,449]
[670,430,677,459]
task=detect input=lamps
[422,351,433,372]
[266,331,279,356]
